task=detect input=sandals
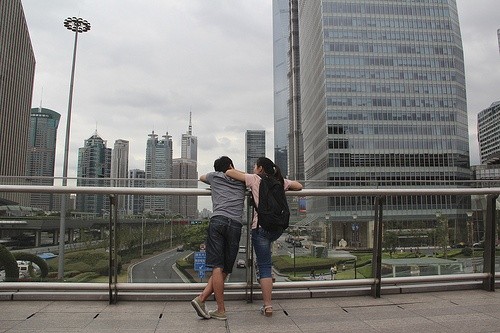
[260,304,272,316]
[256,272,276,279]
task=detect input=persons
[281,243,283,249]
[401,247,417,253]
[310,262,347,280]
[225,157,303,316]
[190,157,249,320]
[277,244,280,249]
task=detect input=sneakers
[208,310,227,320]
[191,296,210,318]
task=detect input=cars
[285,235,294,242]
[473,240,484,247]
[293,240,302,246]
[176,245,184,251]
[451,242,466,248]
[238,246,247,253]
[237,260,246,268]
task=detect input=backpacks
[250,174,290,232]
